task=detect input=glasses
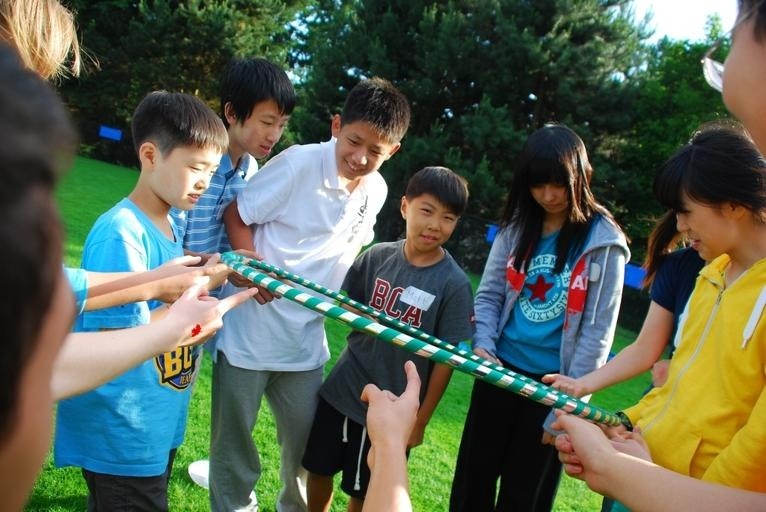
[699,0,766,93]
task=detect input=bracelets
[615,411,634,433]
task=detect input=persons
[299,165,478,512]
[2,45,421,512]
[52,88,263,512]
[549,1,766,512]
[210,76,411,512]
[447,123,632,512]
[1,0,103,83]
[596,118,766,512]
[168,57,295,264]
[542,210,709,512]
